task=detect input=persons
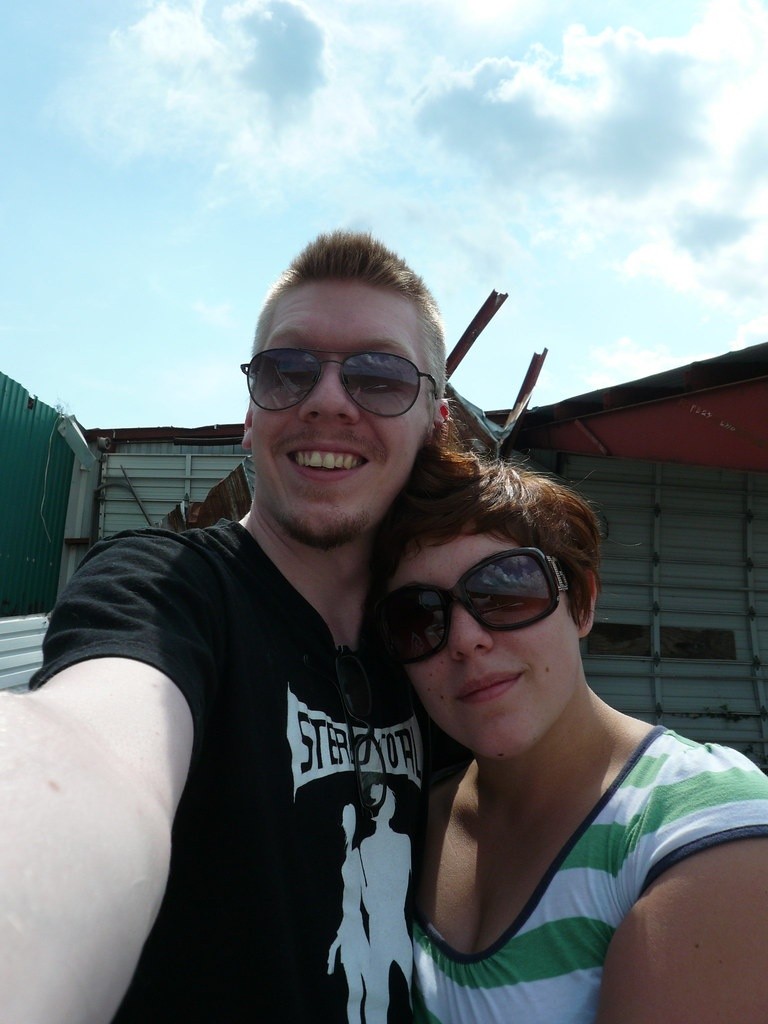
[0,227,474,1024]
[373,443,768,1024]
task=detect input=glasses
[365,548,569,665]
[240,347,437,419]
